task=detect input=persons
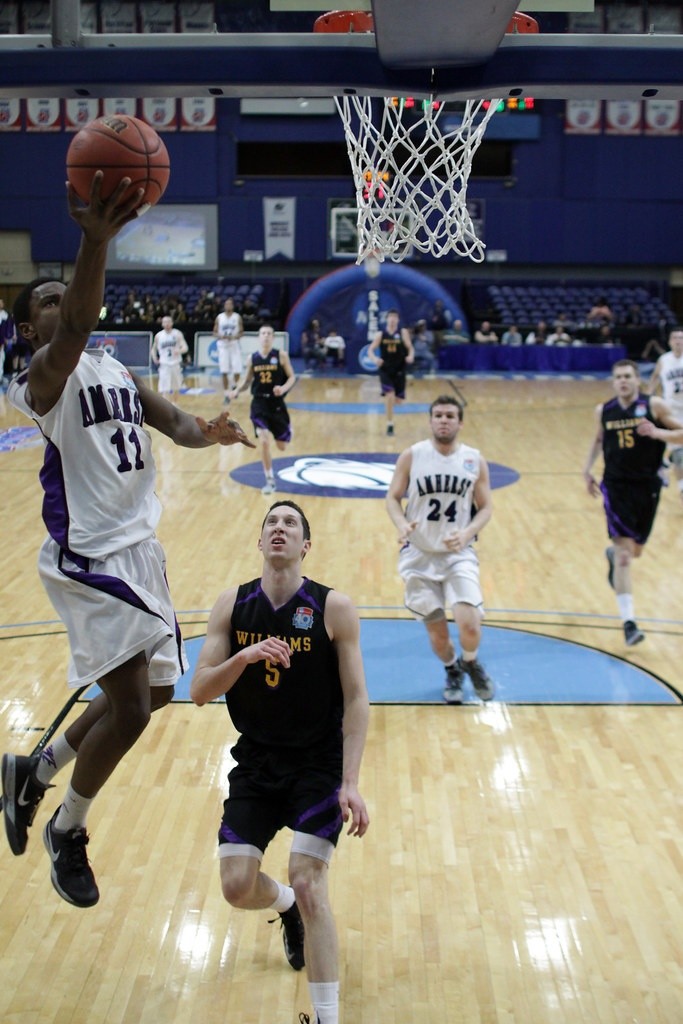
[151,315,188,401]
[0,299,26,384]
[213,299,242,394]
[645,330,683,499]
[384,394,495,707]
[413,300,667,375]
[192,503,368,1023]
[366,310,415,436]
[97,290,256,323]
[300,320,326,371]
[224,322,296,497]
[585,361,683,645]
[323,331,345,369]
[0,168,256,907]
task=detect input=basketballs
[67,114,170,210]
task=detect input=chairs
[102,284,265,322]
[487,283,677,329]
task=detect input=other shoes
[231,384,236,391]
[657,460,670,487]
[387,425,393,436]
[262,480,277,496]
[221,396,230,406]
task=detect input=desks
[436,344,627,378]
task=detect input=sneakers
[268,886,306,971]
[42,803,100,908]
[606,547,616,589]
[1,752,56,856]
[458,652,495,701]
[442,659,464,702]
[624,621,645,646]
